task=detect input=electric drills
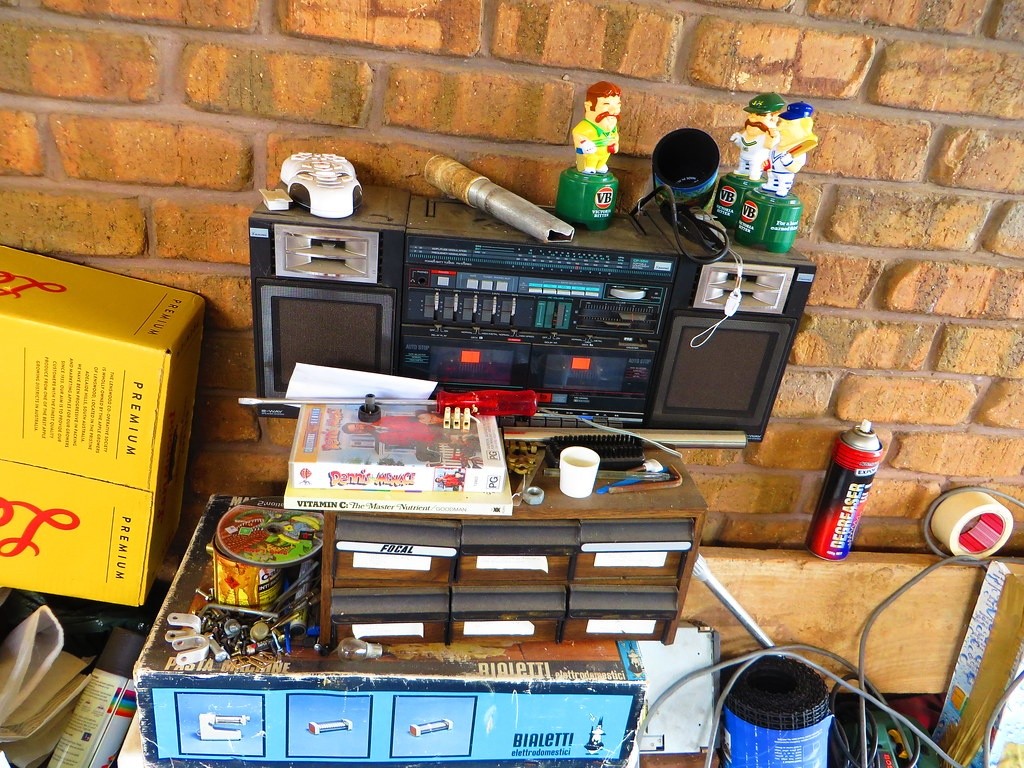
[833,693,941,768]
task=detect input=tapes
[930,491,1014,562]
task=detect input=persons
[343,409,479,445]
[730,91,818,198]
[572,82,622,175]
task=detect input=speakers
[649,211,816,443]
[248,184,413,419]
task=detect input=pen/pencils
[541,467,679,482]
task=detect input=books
[284,403,514,515]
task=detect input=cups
[559,446,600,498]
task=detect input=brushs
[545,435,646,471]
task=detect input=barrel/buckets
[207,533,281,620]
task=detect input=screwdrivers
[236,390,539,418]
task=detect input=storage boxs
[128,493,652,768]
[0,244,208,606]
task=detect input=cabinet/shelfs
[318,443,708,646]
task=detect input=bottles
[807,420,884,561]
[47,626,147,768]
